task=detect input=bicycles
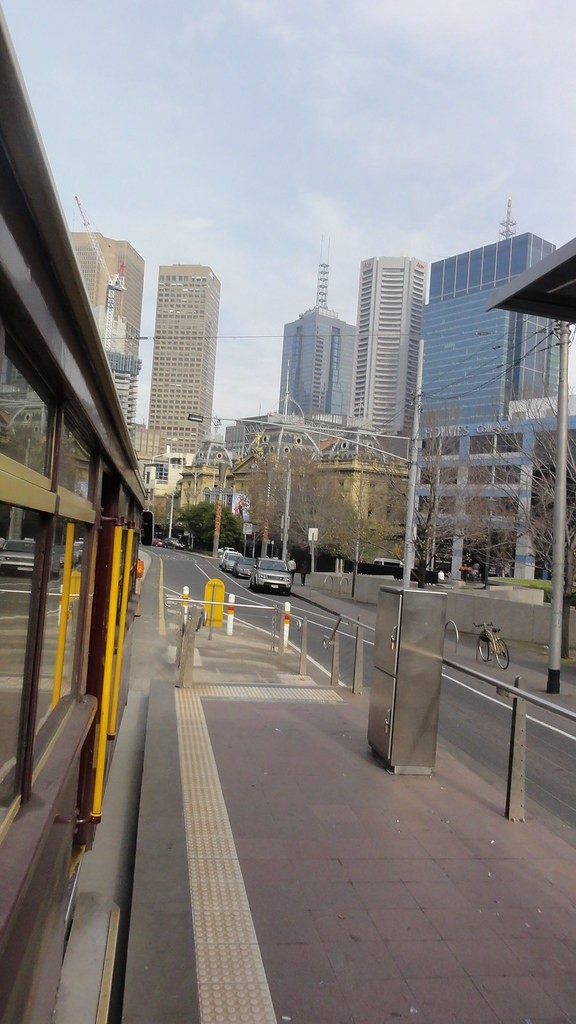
[472,620,510,670]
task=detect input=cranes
[75,195,128,349]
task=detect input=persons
[288,557,296,584]
[300,558,309,586]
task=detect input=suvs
[249,557,294,596]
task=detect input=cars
[0,537,54,574]
[218,546,244,572]
[59,540,85,568]
[153,536,186,550]
[232,556,258,578]
